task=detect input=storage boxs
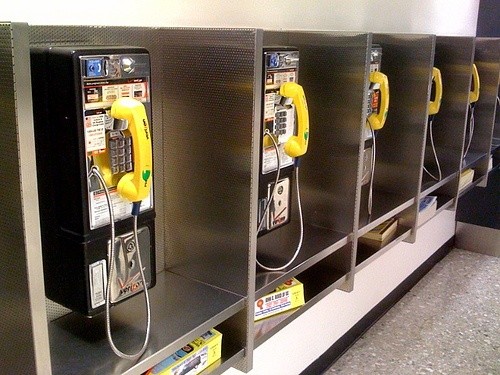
[143,329,223,375]
[253,277,304,322]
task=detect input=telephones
[468,64,480,103]
[359,43,389,185]
[427,67,443,114]
[29,42,158,317]
[255,44,310,239]
